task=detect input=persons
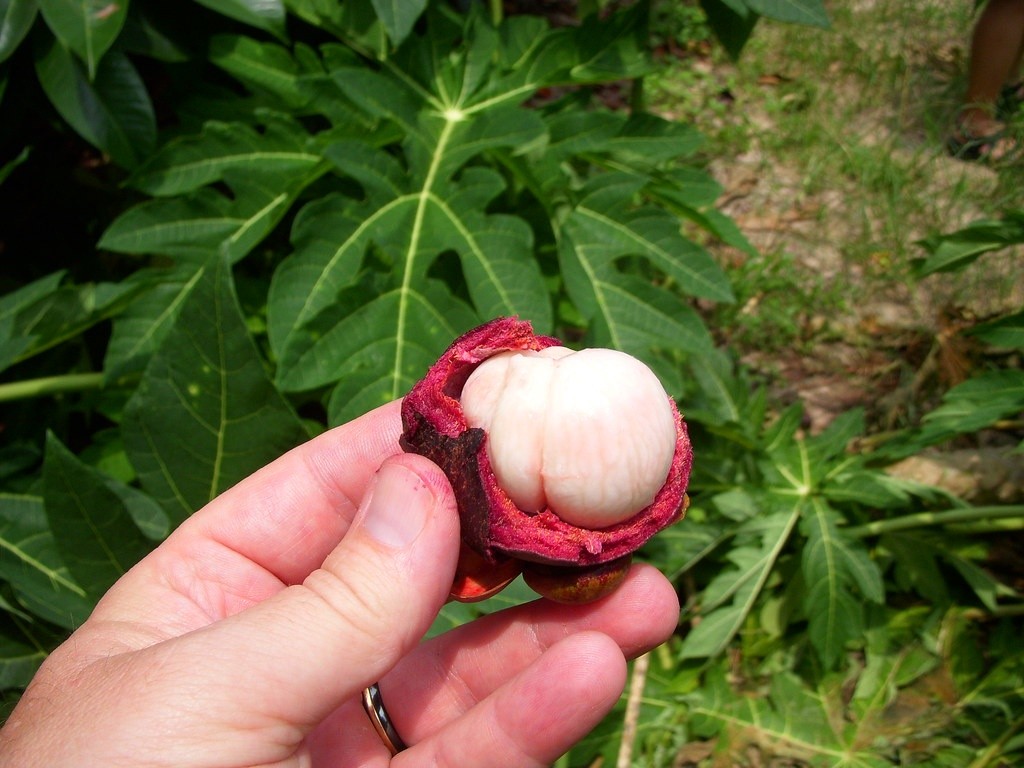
[1,397,680,768]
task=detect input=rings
[360,681,407,758]
